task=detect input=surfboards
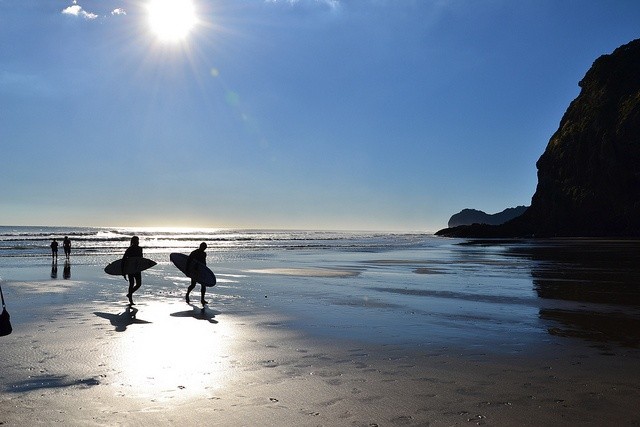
[170,253,215,286]
[104,257,157,275]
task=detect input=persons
[63,236,71,259]
[121,236,143,305]
[185,242,209,304]
[50,239,58,262]
